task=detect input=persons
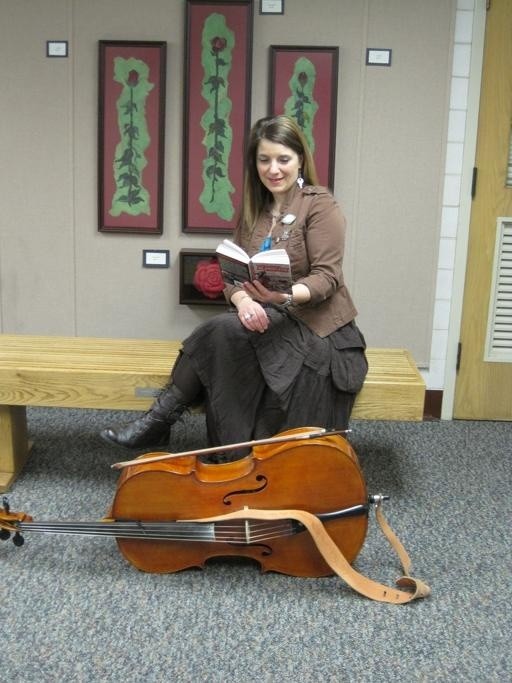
[99,112,370,463]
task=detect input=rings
[244,313,250,323]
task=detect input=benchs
[0,330,426,495]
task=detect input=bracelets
[275,292,293,311]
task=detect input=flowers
[114,69,145,208]
[290,72,311,132]
[193,259,224,299]
[204,37,229,203]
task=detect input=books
[215,238,294,296]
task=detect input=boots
[100,384,191,450]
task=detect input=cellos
[0,422,370,577]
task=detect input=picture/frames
[97,40,167,235]
[178,249,231,305]
[182,0,254,235]
[268,44,339,198]
